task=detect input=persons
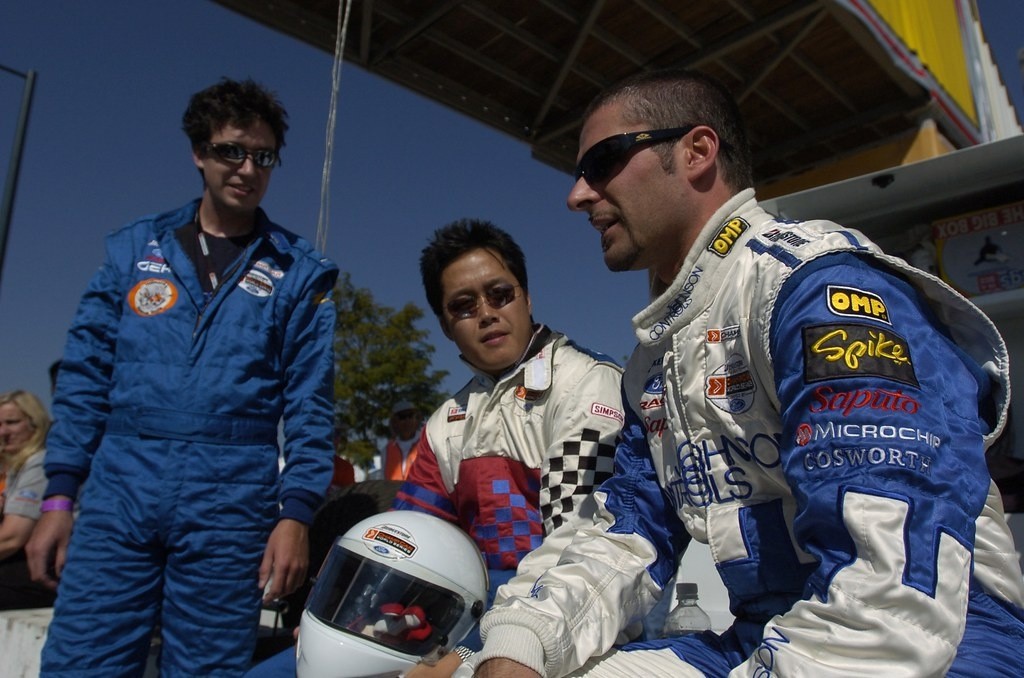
[243,219,673,678]
[0,389,80,612]
[28,77,338,678]
[474,75,1023,678]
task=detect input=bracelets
[39,500,72,511]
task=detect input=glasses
[438,284,527,319]
[575,125,733,189]
[202,142,281,167]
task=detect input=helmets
[296,509,489,678]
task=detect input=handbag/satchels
[0,506,58,612]
